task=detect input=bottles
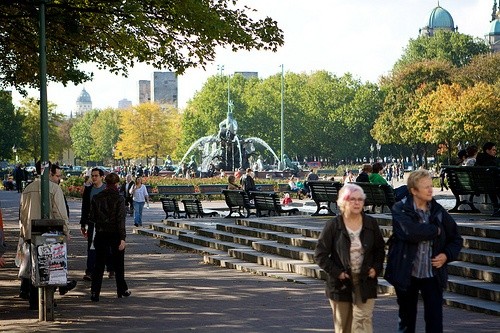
[147,203,149,209]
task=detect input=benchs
[254,183,298,199]
[442,164,500,217]
[220,189,257,218]
[249,191,299,217]
[181,199,219,218]
[349,182,397,214]
[160,197,185,221]
[305,179,341,216]
[147,184,229,200]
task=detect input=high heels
[91,293,99,302]
[118,290,131,298]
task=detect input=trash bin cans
[30,217,68,287]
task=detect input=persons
[120,155,265,227]
[0,160,73,311]
[88,173,131,300]
[383,169,464,333]
[277,154,404,200]
[282,193,292,206]
[439,141,500,216]
[314,183,386,333]
[78,168,114,280]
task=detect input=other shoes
[83,274,94,280]
[59,279,76,295]
[30,301,39,310]
[109,272,116,278]
